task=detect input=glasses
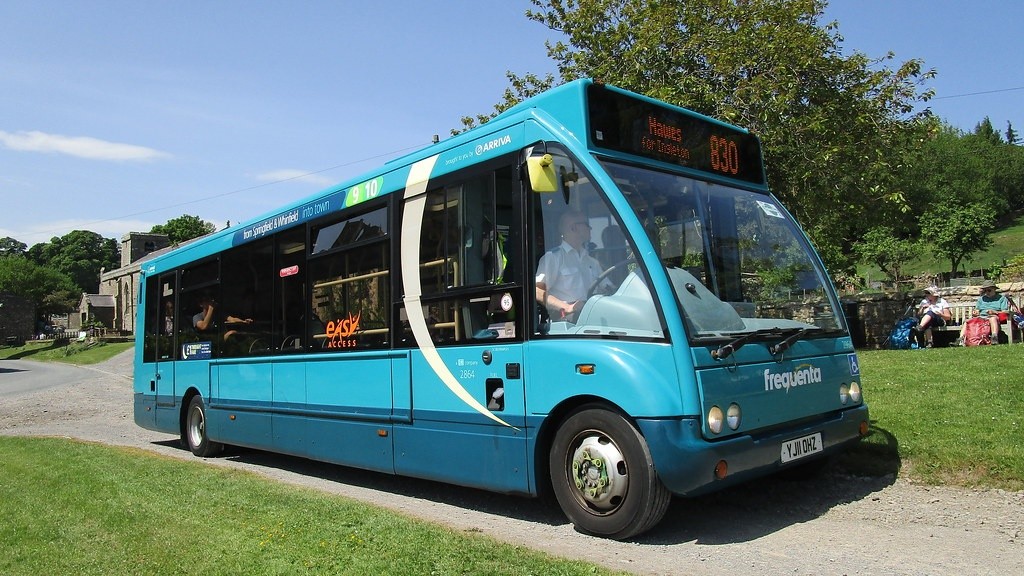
[925,292,930,294]
[571,221,590,229]
[984,289,994,292]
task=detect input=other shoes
[912,325,923,336]
[925,343,932,348]
[949,339,961,347]
[990,334,998,345]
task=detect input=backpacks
[889,317,919,349]
[965,317,991,346]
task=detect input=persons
[949,280,1009,346]
[192,289,254,356]
[913,285,951,348]
[536,209,619,324]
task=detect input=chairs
[248,337,280,354]
[509,209,557,287]
[280,335,304,352]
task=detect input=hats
[980,280,1000,289]
[924,284,941,297]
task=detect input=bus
[134,75,870,541]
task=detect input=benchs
[914,296,1020,348]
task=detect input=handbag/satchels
[1008,297,1024,331]
